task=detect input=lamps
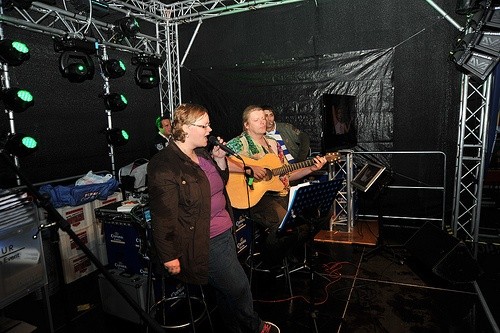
[105,128,130,144]
[5,132,39,160]
[0,87,34,114]
[0,39,32,67]
[131,55,162,89]
[70,0,111,18]
[99,92,127,112]
[102,57,127,78]
[115,16,140,39]
[55,32,99,83]
[448,0,500,81]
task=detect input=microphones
[209,136,238,158]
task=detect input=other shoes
[260,321,280,333]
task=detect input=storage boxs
[57,192,123,282]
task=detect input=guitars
[225,152,342,210]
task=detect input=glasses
[187,121,211,129]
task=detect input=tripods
[274,179,343,283]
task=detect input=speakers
[406,221,478,290]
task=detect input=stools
[241,210,298,318]
[131,203,218,333]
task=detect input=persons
[147,103,282,333]
[261,104,310,186]
[223,105,327,281]
[159,117,173,149]
[331,103,354,134]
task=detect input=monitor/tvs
[319,93,358,155]
